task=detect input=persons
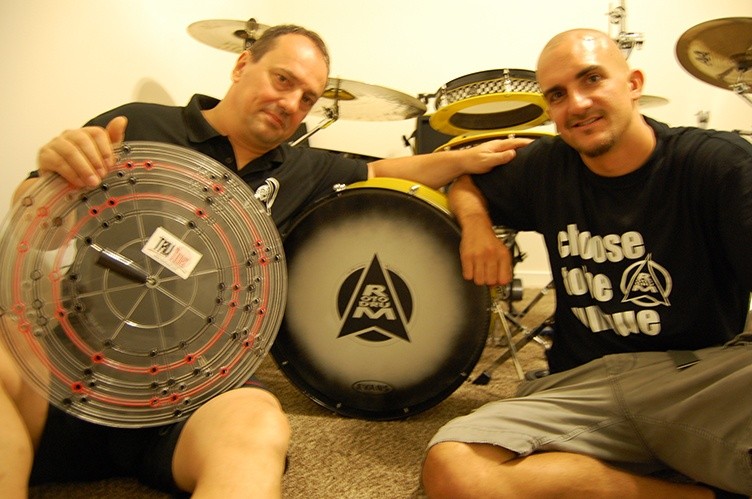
[418,29,752,499]
[0,24,536,499]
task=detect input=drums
[268,177,499,423]
[431,128,560,154]
[413,92,518,239]
[429,68,551,137]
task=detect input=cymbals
[674,16,752,94]
[308,77,427,123]
[186,18,272,53]
[636,95,669,109]
